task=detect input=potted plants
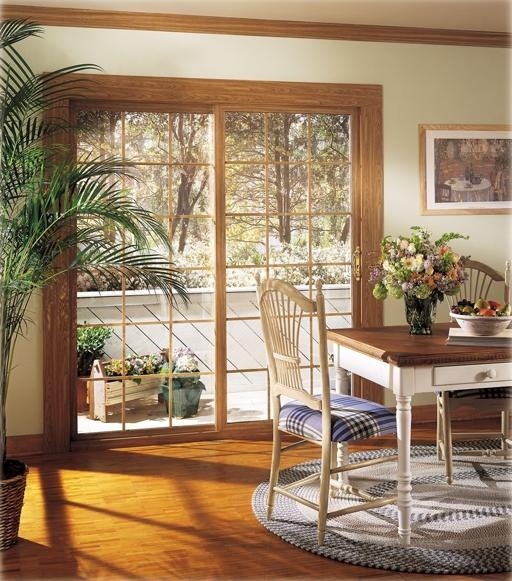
[0,16,192,548]
[75,325,113,410]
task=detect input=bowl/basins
[449,311,512,335]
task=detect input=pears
[474,296,490,310]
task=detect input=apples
[489,301,501,311]
[480,309,496,315]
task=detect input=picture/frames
[418,125,512,217]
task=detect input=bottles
[465,163,472,179]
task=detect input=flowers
[108,343,206,394]
[367,226,472,304]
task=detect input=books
[445,326,512,347]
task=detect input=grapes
[451,299,475,314]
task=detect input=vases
[163,387,203,420]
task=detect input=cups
[449,175,472,188]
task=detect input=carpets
[250,435,512,575]
